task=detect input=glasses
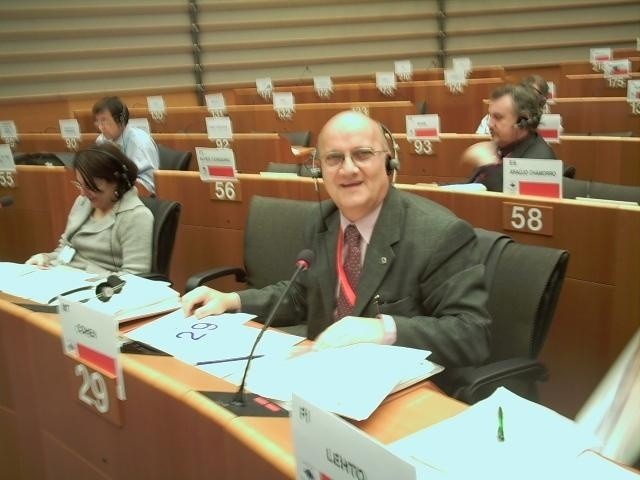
[69,180,95,191]
[317,147,388,167]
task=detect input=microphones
[229,249,317,407]
[313,176,329,233]
[0,197,12,209]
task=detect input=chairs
[1,130,640,406]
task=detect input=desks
[1,298,470,478]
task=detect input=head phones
[530,83,546,107]
[85,148,129,185]
[46,275,126,307]
[116,102,127,123]
[516,105,542,130]
[310,120,401,178]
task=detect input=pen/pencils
[497,406,504,441]
[197,355,264,365]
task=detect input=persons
[460,74,556,196]
[92,97,160,199]
[24,143,154,277]
[183,111,492,400]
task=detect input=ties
[337,224,361,320]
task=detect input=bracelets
[377,312,396,346]
